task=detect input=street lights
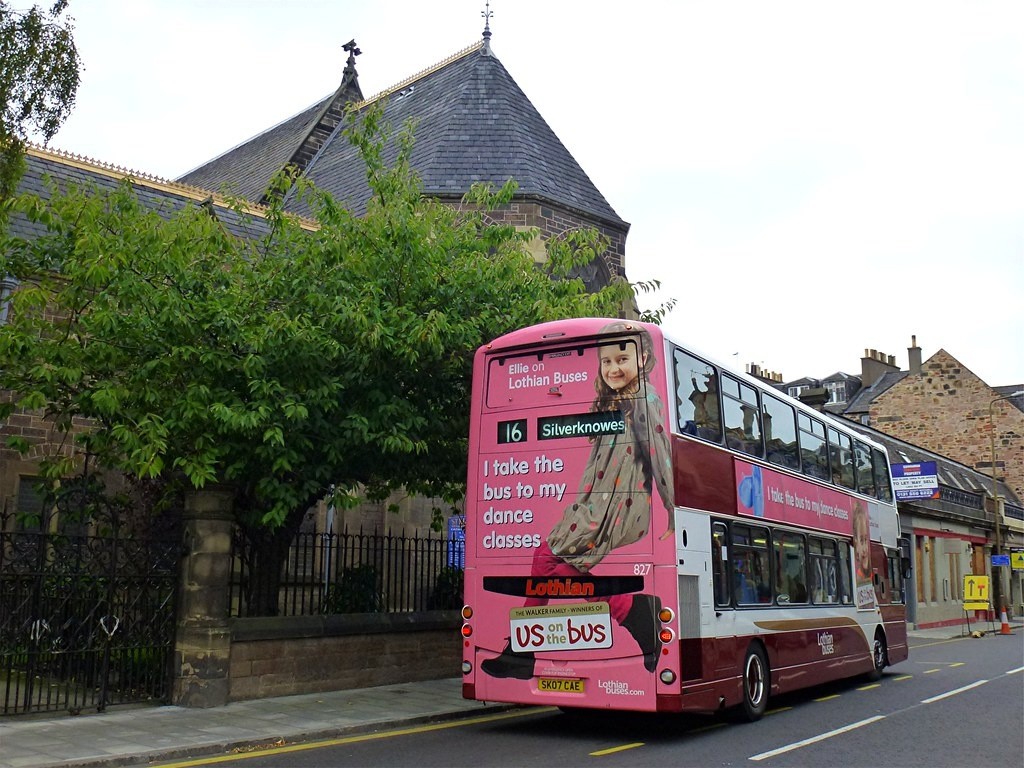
[989,389,1023,623]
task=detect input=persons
[854,500,874,609]
[481,322,675,680]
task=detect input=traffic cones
[995,605,1016,635]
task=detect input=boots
[481,636,536,680]
[619,593,662,674]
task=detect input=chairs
[733,567,750,604]
[745,579,760,603]
[680,417,700,437]
[743,441,761,457]
[726,430,746,452]
[698,420,720,443]
[768,445,826,479]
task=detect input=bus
[461,316,909,719]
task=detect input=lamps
[1008,533,1015,539]
[925,542,930,552]
[965,547,973,554]
